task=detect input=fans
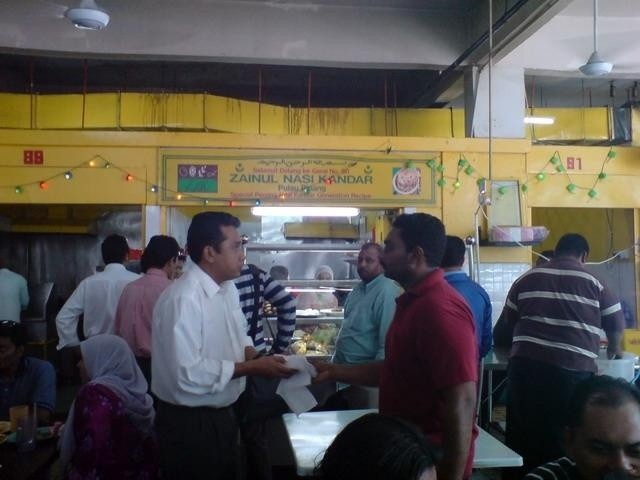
[578,0,614,81]
[65,0,110,32]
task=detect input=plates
[296,309,320,317]
[320,308,344,316]
[0,421,54,444]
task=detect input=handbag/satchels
[249,374,281,401]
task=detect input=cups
[9,405,28,432]
[15,416,37,452]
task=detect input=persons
[310,412,444,480]
[1,232,179,479]
[523,373,640,479]
[437,235,492,360]
[269,266,290,280]
[298,265,338,331]
[313,213,480,480]
[492,232,625,479]
[148,210,298,479]
[329,242,402,408]
[228,232,296,480]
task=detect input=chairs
[23,282,55,355]
